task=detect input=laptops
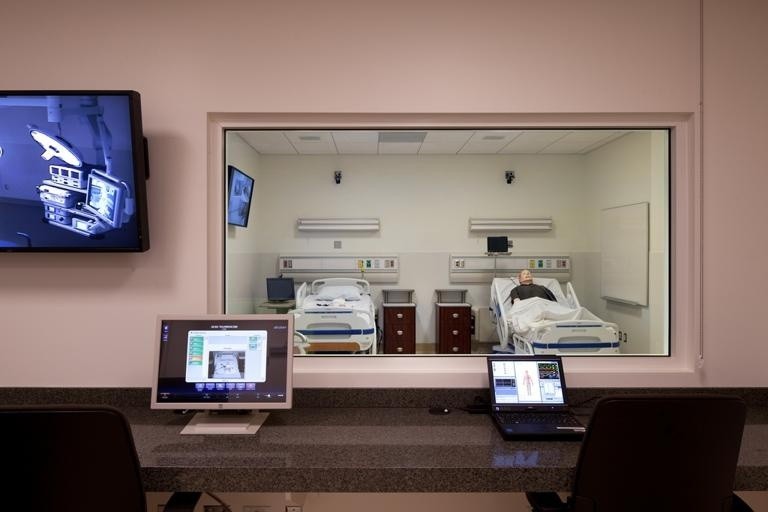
[488,355,587,440]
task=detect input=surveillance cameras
[335,171,342,184]
[505,170,515,183]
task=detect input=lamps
[27,95,82,168]
[471,218,552,231]
[297,218,380,233]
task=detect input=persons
[510,270,558,305]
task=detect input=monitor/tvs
[0,91,150,253]
[228,165,255,227]
[150,312,294,435]
[267,277,295,304]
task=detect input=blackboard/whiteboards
[600,202,649,308]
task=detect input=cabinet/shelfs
[41,179,124,239]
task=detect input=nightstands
[435,289,471,354]
[382,289,416,354]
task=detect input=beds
[490,277,620,355]
[287,277,377,354]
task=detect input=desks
[258,300,296,314]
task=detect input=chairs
[525,392,757,511]
[1,404,203,511]
